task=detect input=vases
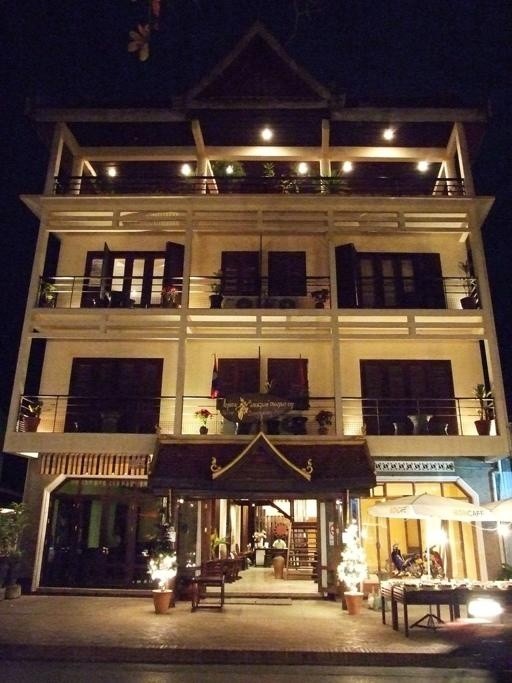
[200,422,207,433]
[320,423,331,435]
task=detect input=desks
[254,547,288,566]
[379,577,508,642]
[407,413,434,434]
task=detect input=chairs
[272,538,286,548]
[191,542,252,612]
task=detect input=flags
[292,354,307,399]
[210,350,229,397]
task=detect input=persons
[270,536,287,549]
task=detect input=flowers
[316,409,333,423]
[195,406,211,425]
[252,528,267,542]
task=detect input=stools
[392,421,405,434]
[436,422,449,434]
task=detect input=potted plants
[470,379,496,434]
[0,501,32,598]
[19,398,46,431]
[311,288,329,308]
[335,519,366,617]
[208,270,225,307]
[41,280,58,308]
[147,518,177,612]
[458,256,477,309]
